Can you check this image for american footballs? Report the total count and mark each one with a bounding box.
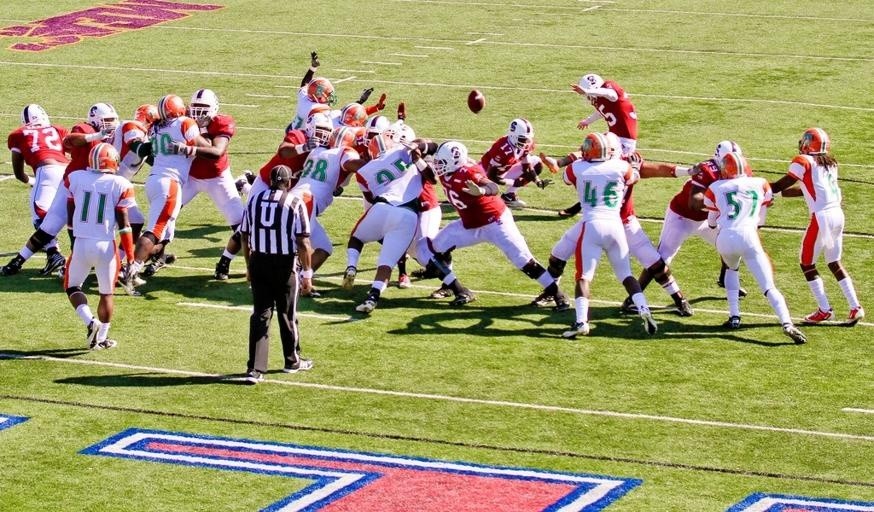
[469,90,484,115]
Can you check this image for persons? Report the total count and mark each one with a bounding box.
[1,52,749,338]
[704,152,808,345]
[769,127,864,328]
[63,142,135,350]
[240,164,313,384]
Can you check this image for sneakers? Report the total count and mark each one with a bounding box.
[559,210,572,216]
[501,192,526,208]
[234,171,254,193]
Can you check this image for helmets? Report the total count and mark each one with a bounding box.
[579,74,605,91]
[87,104,117,134]
[21,104,50,128]
[509,119,532,151]
[581,132,621,162]
[712,140,754,177]
[133,89,219,132]
[88,142,119,173]
[799,128,829,155]
[307,77,469,172]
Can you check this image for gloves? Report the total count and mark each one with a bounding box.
[463,179,487,198]
[168,139,197,157]
[310,51,319,71]
[333,187,344,197]
[536,179,554,188]
[687,162,709,178]
[98,128,115,140]
[628,152,641,169]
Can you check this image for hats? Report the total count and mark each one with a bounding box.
[270,165,297,181]
